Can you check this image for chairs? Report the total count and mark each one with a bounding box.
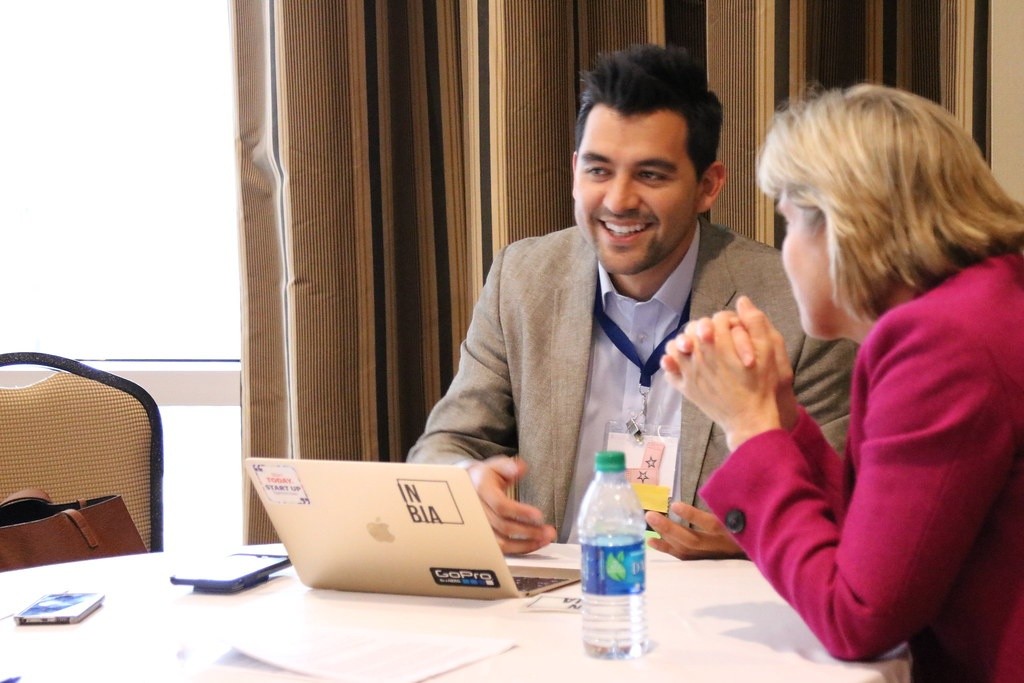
[0,351,165,551]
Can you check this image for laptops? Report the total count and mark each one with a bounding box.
[245,456,582,600]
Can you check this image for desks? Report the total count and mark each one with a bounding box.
[0,543,914,683]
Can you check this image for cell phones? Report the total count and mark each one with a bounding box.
[14,591,107,626]
[171,553,292,594]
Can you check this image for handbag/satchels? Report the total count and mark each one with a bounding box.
[0,489,148,573]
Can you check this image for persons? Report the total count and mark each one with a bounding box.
[406,43,858,562]
[660,85,1023,683]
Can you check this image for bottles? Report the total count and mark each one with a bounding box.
[576,450,651,661]
